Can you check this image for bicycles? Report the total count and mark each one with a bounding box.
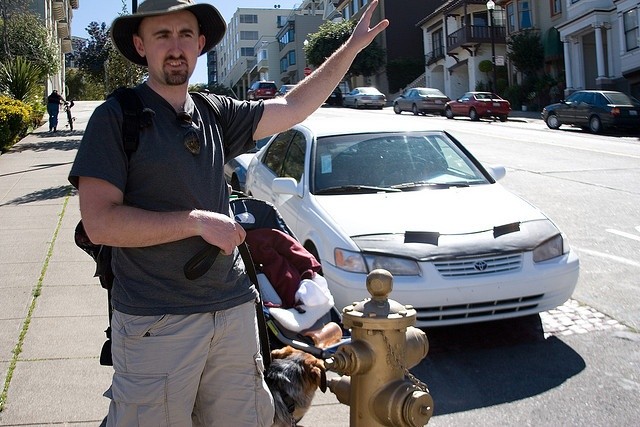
[59,101,76,131]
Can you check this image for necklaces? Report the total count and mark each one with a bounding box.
[178,110,201,154]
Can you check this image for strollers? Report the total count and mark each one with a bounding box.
[224,190,350,376]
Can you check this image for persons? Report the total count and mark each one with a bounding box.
[47,88,66,132]
[68,1,389,427]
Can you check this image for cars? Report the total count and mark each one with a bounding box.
[324,268,434,426]
[322,86,343,107]
[247,81,277,101]
[444,92,511,122]
[275,85,297,100]
[393,88,450,116]
[541,91,640,134]
[246,119,579,336]
[342,87,386,110]
[222,130,279,198]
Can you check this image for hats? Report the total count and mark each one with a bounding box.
[109,0,227,66]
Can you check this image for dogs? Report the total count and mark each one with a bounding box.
[100,336,327,427]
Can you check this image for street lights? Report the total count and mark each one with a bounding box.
[486,0,499,95]
